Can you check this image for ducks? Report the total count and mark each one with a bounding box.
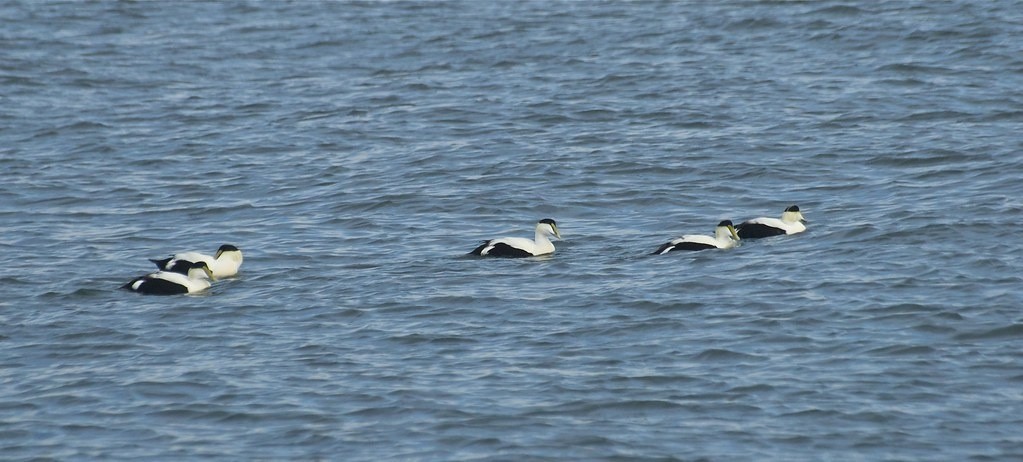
[649,205,807,256]
[469,218,561,257]
[115,244,243,292]
